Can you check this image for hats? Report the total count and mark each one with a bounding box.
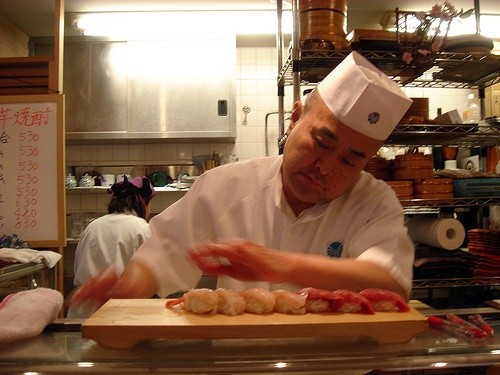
[317,51,414,141]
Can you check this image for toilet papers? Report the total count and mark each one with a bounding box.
[409,217,466,250]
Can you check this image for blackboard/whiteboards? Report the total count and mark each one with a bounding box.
[0,93,68,248]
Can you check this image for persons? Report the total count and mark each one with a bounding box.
[66,176,156,318]
[69,52,416,317]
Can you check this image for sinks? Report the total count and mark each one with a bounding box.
[167,181,193,189]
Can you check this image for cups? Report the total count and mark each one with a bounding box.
[99,174,115,186]
[70,212,97,239]
[151,170,167,187]
[117,174,130,184]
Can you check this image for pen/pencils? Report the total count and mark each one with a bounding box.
[428,314,495,340]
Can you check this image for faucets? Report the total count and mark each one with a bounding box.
[179,172,190,177]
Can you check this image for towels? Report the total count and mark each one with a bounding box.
[1,245,62,270]
[0,286,66,341]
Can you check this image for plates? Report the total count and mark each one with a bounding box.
[457,229,500,280]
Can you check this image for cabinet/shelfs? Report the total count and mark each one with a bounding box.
[29,32,237,142]
[276,0,500,289]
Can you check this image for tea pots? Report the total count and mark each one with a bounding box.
[79,173,97,187]
[65,173,77,188]
